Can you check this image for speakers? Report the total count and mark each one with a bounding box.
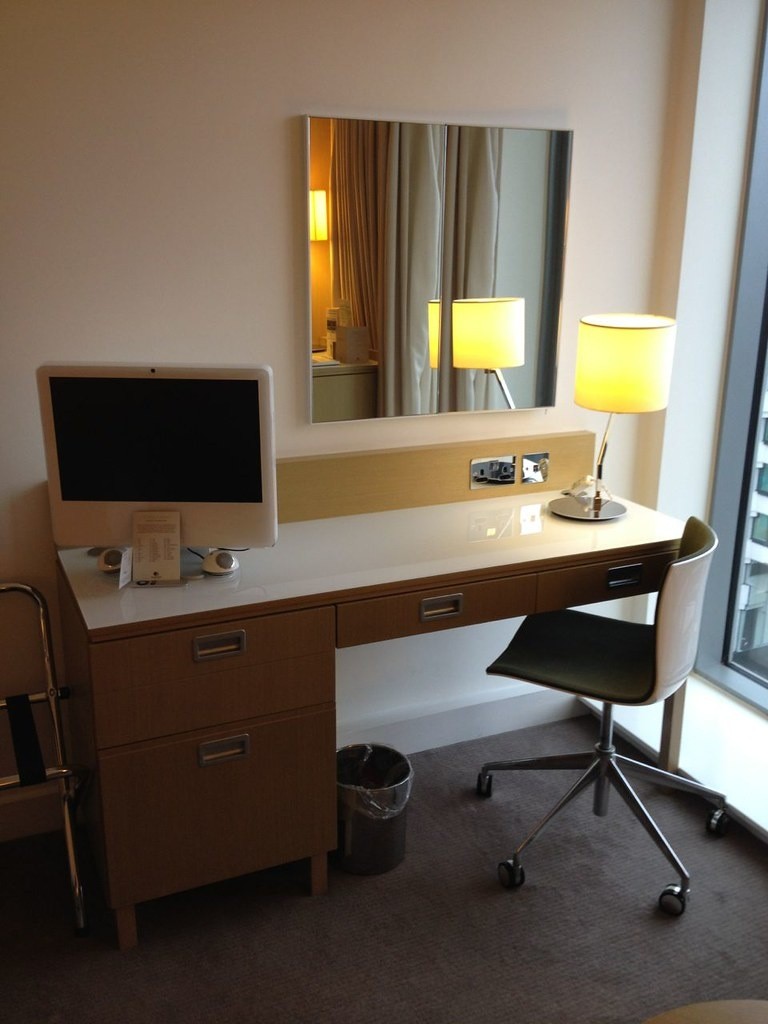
[200,549,239,576]
[98,548,124,574]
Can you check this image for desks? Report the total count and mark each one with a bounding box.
[49,538,697,954]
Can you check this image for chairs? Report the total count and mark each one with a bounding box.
[474,516,730,917]
[0,582,83,938]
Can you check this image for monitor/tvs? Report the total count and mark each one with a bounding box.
[36,363,279,580]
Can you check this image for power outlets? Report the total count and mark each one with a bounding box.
[469,451,548,491]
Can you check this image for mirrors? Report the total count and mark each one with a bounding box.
[303,117,572,428]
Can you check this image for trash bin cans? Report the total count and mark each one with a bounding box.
[335,743,415,876]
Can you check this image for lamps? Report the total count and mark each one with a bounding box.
[550,312,679,522]
[453,299,534,412]
